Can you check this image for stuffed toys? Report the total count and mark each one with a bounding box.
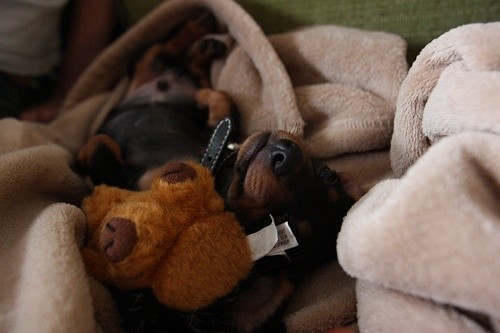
[82,161,253,311]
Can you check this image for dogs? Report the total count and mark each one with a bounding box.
[73,9,360,286]
